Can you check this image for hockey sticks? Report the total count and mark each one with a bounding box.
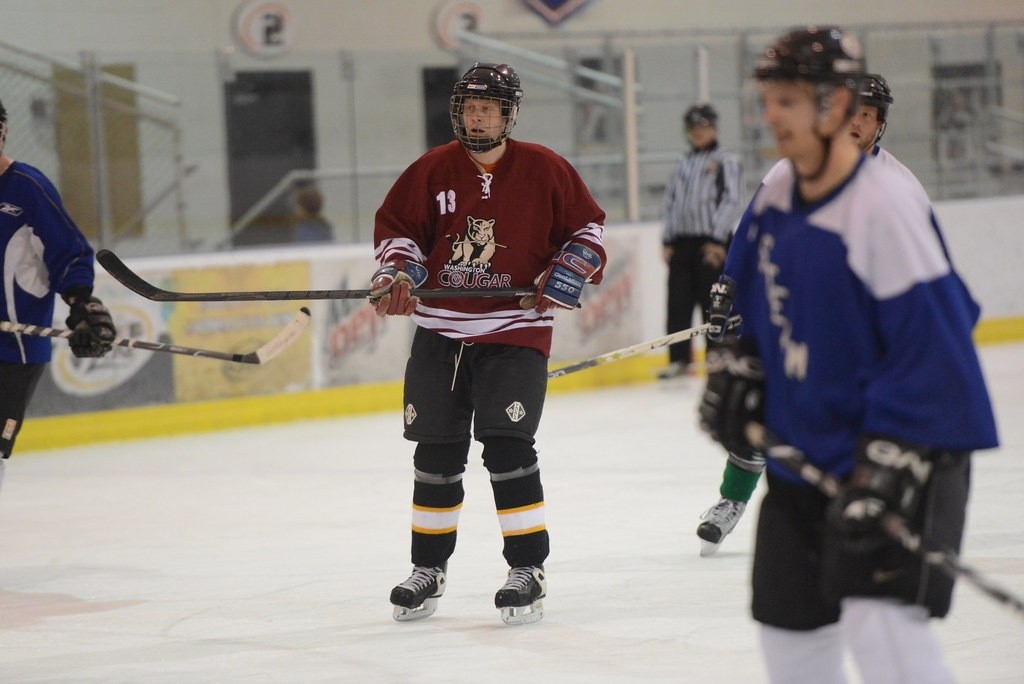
[0,308,311,366]
[548,314,736,380]
[740,412,1024,623]
[95,247,578,307]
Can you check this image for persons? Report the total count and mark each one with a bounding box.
[293,188,332,243]
[701,25,1001,684]
[698,74,981,553]
[0,100,117,485]
[370,62,608,624]
[659,103,743,379]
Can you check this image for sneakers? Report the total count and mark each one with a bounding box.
[390,562,447,621]
[697,498,746,555]
[495,566,547,626]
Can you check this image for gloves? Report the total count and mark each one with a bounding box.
[65,297,116,359]
[531,242,601,314]
[368,259,427,317]
[662,246,672,263]
[696,357,764,444]
[840,438,931,543]
[704,243,726,266]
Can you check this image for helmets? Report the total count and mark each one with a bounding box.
[0,102,8,151]
[858,73,894,106]
[747,24,867,86]
[449,63,524,152]
[684,103,718,126]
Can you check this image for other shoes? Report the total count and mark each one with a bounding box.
[659,362,686,378]
